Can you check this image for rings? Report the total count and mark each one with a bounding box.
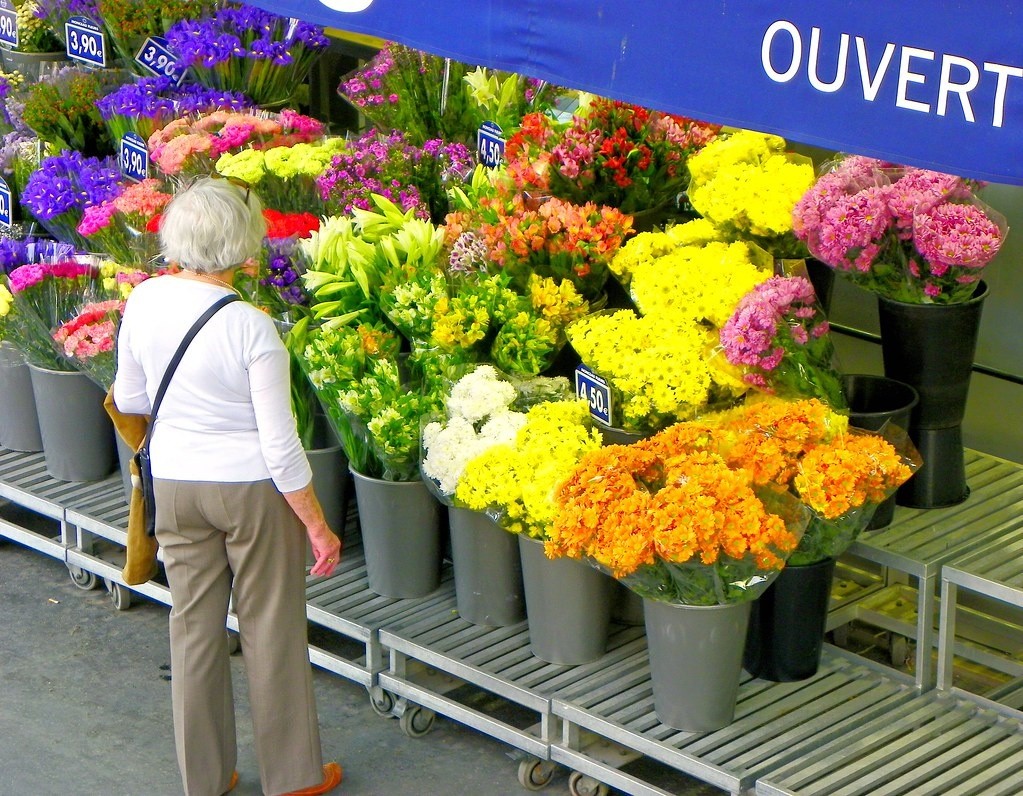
[325,559,334,563]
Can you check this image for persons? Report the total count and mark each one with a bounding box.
[114,175,341,796]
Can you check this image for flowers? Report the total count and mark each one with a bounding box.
[686,129,814,259]
[682,393,924,568]
[791,151,1008,305]
[454,396,606,541]
[717,275,849,412]
[563,307,712,431]
[419,366,528,503]
[542,421,814,607]
[0,0,719,479]
[607,217,773,403]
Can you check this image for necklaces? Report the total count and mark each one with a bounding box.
[183,267,245,301]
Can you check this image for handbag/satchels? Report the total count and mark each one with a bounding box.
[133,446,155,535]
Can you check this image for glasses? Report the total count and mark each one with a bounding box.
[187,172,251,208]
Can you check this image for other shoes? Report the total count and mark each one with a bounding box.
[225,769,238,792]
[278,762,342,796]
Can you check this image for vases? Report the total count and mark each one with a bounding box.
[112,424,138,506]
[642,597,753,733]
[75,58,128,79]
[0,341,44,452]
[893,425,971,510]
[347,459,445,598]
[447,507,527,627]
[777,256,836,320]
[518,533,615,665]
[1,47,73,79]
[610,580,648,626]
[739,556,836,684]
[837,374,919,532]
[128,66,145,82]
[84,250,117,261]
[876,280,992,430]
[25,360,115,481]
[18,232,57,246]
[260,92,299,113]
[302,413,348,565]
[271,318,322,336]
